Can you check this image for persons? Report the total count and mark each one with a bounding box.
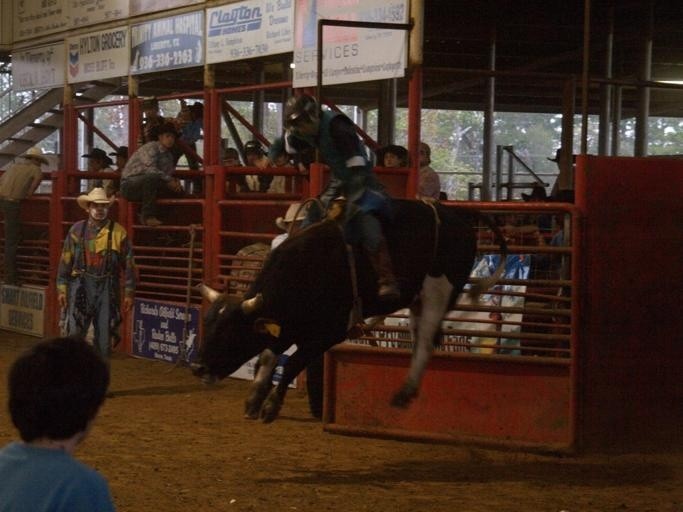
[376,143,408,169]
[0,332,116,511]
[280,92,404,303]
[546,148,577,197]
[56,187,139,398]
[464,185,576,304]
[0,147,50,285]
[419,142,442,200]
[119,121,185,227]
[80,95,310,197]
[269,201,309,252]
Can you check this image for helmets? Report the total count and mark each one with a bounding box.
[282,93,317,126]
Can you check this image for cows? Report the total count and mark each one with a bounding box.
[188,193,508,425]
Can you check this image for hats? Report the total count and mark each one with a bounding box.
[77,187,116,209]
[156,122,179,138]
[109,146,129,158]
[81,148,113,165]
[374,145,409,163]
[20,148,49,166]
